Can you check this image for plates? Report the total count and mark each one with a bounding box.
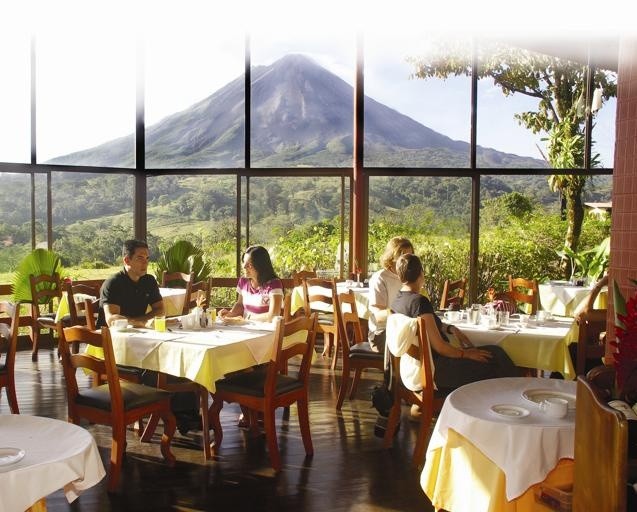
[489,403,531,418]
[0,446,25,468]
[521,387,577,410]
[548,280,570,285]
[129,331,188,342]
[249,322,275,332]
[110,325,134,331]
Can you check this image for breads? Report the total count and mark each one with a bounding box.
[166,319,178,323]
[224,316,243,324]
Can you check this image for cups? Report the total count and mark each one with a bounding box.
[539,396,569,419]
[111,319,127,329]
[570,274,588,285]
[443,304,554,330]
[179,306,217,328]
[154,315,165,331]
[345,279,353,287]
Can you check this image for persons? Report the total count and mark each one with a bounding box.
[390,253,520,388]
[83,239,204,436]
[216,245,285,427]
[366,235,415,353]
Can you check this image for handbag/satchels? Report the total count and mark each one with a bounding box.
[374,415,401,437]
[481,300,515,316]
[370,385,401,417]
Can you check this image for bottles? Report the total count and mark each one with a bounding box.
[363,279,370,288]
[63,276,71,291]
